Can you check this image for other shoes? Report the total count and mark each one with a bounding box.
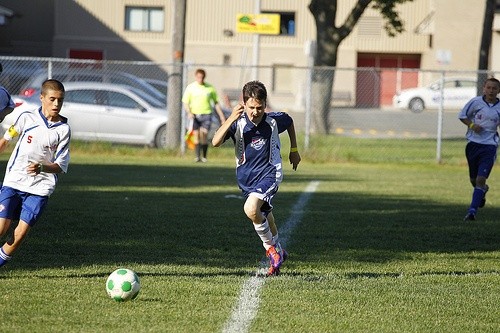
[194,158,207,163]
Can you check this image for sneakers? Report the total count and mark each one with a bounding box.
[464,211,476,221]
[479,184,489,208]
[266,242,289,277]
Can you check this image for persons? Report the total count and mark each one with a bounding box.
[0,79,71,269]
[211,80,302,277]
[0,62,15,123]
[458,78,500,220]
[181,68,226,163]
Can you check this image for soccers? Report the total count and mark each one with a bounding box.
[106,268,141,302]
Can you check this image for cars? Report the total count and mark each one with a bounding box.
[11,80,220,150]
[393,75,478,113]
[19,67,168,104]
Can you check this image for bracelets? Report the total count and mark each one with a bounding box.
[39,163,44,172]
[290,147,298,152]
[469,123,475,129]
